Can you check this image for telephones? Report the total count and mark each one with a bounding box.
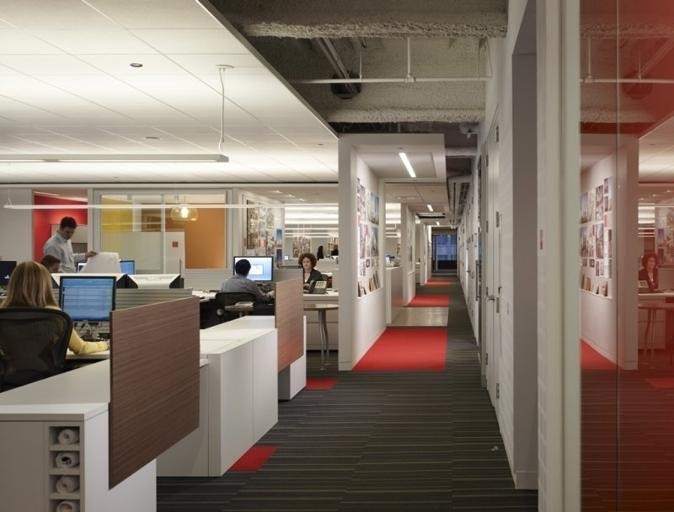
[308,280,327,294]
[638,279,652,294]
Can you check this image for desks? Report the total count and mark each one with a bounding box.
[633,301,674,375]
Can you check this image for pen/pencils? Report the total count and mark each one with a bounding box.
[74,321,85,334]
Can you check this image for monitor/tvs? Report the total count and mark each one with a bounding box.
[78,263,86,272]
[60,275,117,323]
[233,256,274,282]
[0,261,17,279]
[119,260,135,275]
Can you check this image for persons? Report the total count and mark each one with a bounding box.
[316,245,324,261]
[39,254,61,273]
[295,252,323,292]
[330,245,338,256]
[215,258,275,303]
[657,248,670,261]
[0,259,111,356]
[639,253,661,292]
[42,216,97,273]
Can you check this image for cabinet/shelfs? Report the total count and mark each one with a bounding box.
[638,290,673,354]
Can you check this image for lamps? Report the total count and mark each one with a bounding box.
[0,62,238,168]
[170,192,199,224]
